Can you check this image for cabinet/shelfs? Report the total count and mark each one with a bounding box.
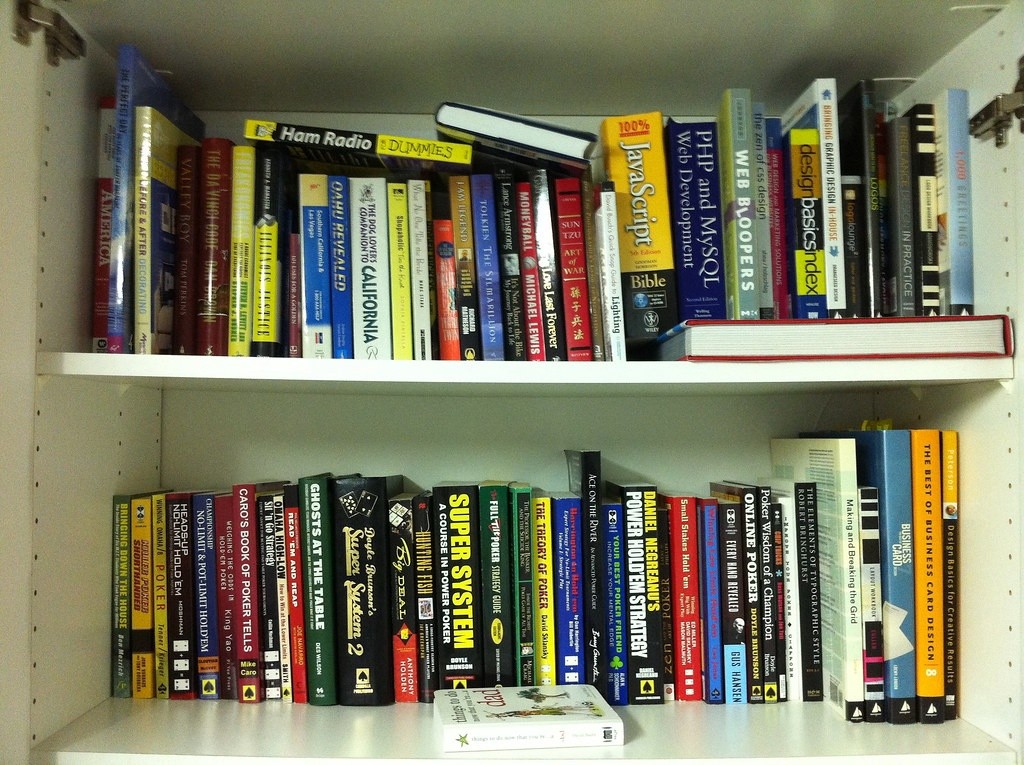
[0,0,1024,765]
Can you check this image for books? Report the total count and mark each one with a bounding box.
[91,44,1013,753]
